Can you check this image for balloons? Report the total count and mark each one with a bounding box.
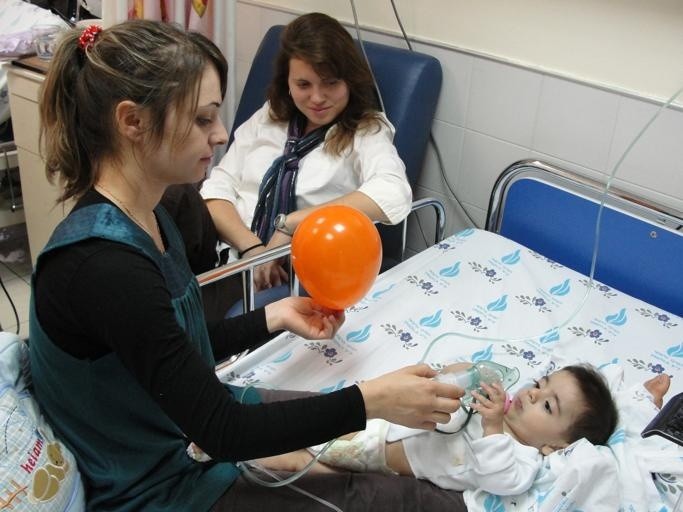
[292,206,383,314]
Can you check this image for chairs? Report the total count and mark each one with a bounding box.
[223,21,444,321]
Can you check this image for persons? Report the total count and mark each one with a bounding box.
[236,364,618,496]
[27,21,670,512]
[162,12,413,327]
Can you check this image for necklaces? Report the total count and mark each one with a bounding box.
[93,179,168,254]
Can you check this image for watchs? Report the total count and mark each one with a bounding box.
[273,212,294,237]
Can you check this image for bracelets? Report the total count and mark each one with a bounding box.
[237,242,265,257]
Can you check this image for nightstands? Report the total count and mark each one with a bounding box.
[10,67,84,272]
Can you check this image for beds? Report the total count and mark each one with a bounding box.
[195,160,683,512]
